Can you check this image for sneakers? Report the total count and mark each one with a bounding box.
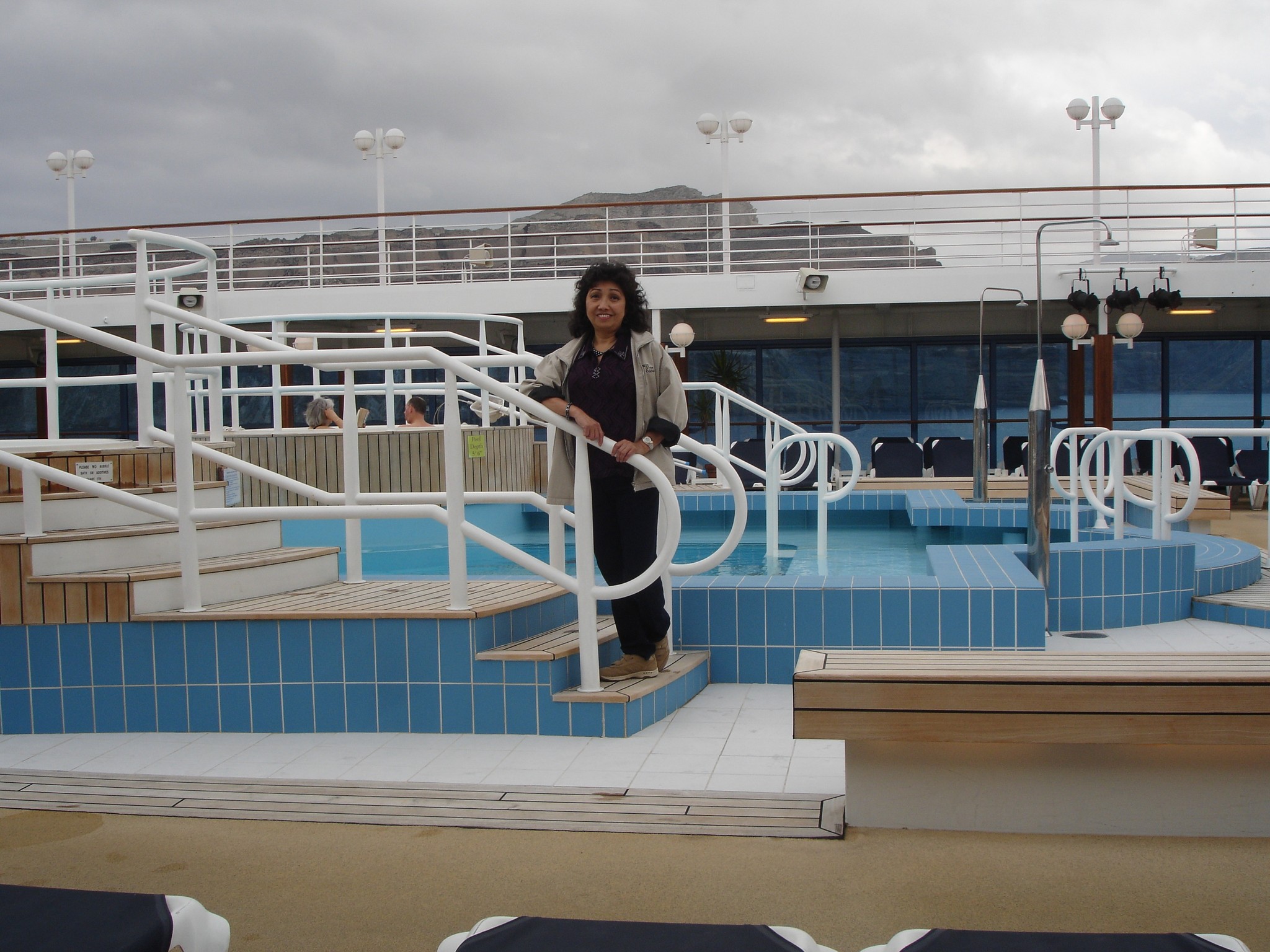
[599,654,659,681]
[654,633,670,672]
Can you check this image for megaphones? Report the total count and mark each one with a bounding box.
[469,244,493,267]
[177,287,204,311]
[796,268,829,294]
[1193,225,1218,250]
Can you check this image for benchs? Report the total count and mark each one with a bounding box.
[795,647,1270,839]
[1123,477,1231,531]
[839,478,1118,497]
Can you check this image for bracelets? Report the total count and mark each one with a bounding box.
[565,403,572,421]
[335,418,341,424]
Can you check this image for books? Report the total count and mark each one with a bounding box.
[356,407,369,428]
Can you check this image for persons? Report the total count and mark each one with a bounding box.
[306,395,436,430]
[518,259,688,680]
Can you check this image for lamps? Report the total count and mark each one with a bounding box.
[1112,313,1144,349]
[1104,267,1141,315]
[1147,266,1182,310]
[1067,268,1101,312]
[367,320,417,332]
[292,338,311,366]
[1181,225,1217,254]
[246,342,271,367]
[1060,314,1093,350]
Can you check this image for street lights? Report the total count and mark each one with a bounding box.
[351,127,406,285]
[696,111,752,274]
[1064,94,1125,265]
[45,147,95,297]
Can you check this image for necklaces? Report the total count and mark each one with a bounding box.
[593,340,617,356]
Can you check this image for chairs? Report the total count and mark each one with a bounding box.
[1004,435,1270,515]
[871,436,976,479]
[673,452,696,485]
[0,889,1252,952]
[730,439,835,492]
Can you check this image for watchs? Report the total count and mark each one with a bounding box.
[640,435,654,451]
